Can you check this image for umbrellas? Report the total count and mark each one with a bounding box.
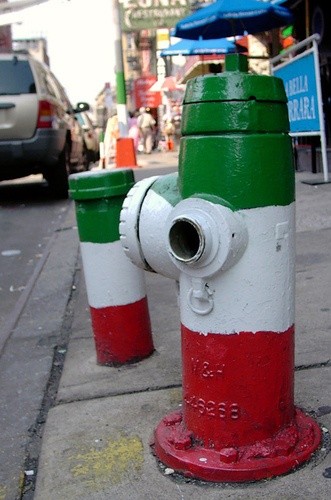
[161,35,249,77]
[170,1,295,54]
[150,77,191,92]
[176,54,227,85]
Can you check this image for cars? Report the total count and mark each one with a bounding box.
[77,111,100,162]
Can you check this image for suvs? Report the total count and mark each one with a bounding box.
[0,52,91,196]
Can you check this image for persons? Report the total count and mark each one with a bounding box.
[104,112,120,168]
[129,111,143,154]
[137,107,158,155]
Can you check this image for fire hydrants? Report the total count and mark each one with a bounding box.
[118,53,322,481]
